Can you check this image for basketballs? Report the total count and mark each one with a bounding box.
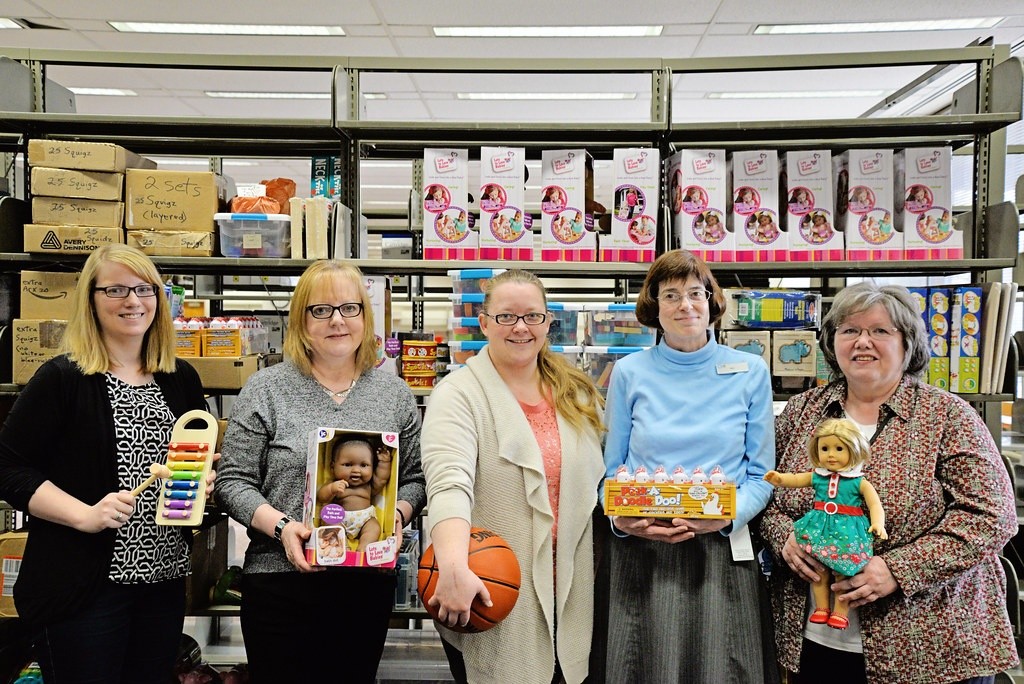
[418,526,520,634]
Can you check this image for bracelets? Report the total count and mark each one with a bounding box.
[395,507,406,528]
[274,516,297,542]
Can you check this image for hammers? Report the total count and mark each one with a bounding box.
[129,463,172,495]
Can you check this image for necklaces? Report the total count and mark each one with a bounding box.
[310,370,355,398]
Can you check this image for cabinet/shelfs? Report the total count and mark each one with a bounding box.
[0,42,1024,684]
[952,56,1024,659]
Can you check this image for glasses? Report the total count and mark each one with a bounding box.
[834,322,903,340]
[305,300,364,319]
[656,289,712,306]
[94,284,160,298]
[479,311,548,325]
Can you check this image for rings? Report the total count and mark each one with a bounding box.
[117,512,123,519]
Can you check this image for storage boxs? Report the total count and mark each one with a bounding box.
[25,139,292,258]
[362,274,400,378]
[447,270,657,390]
[0,530,28,618]
[719,288,983,393]
[302,427,399,569]
[605,475,737,519]
[392,530,419,611]
[218,416,228,454]
[423,147,964,261]
[14,269,279,389]
[186,516,229,609]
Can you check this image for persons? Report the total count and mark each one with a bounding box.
[480,183,523,239]
[904,184,950,237]
[424,185,466,239]
[215,261,428,684]
[419,271,610,684]
[0,241,223,684]
[598,246,776,684]
[316,437,393,553]
[680,188,725,243]
[762,419,888,630]
[757,283,1021,684]
[622,187,655,242]
[735,187,778,244]
[849,188,892,240]
[788,187,833,242]
[321,529,342,558]
[542,187,585,241]
